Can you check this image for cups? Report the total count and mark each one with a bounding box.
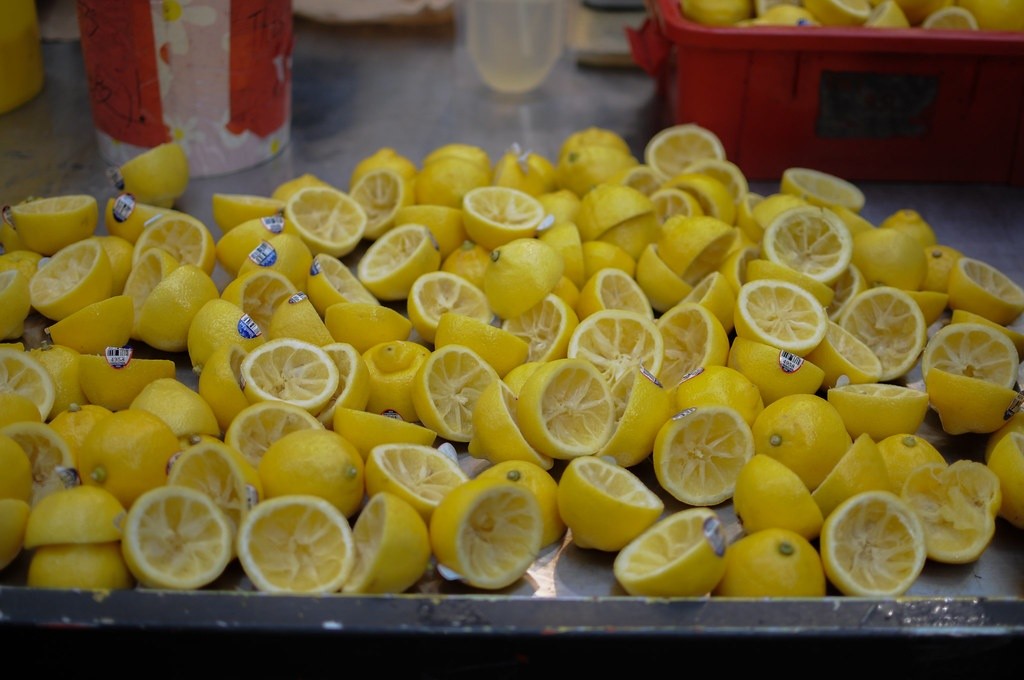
[79,0,292,178]
[467,0,561,92]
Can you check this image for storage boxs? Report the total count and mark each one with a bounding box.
[639,0,1024,184]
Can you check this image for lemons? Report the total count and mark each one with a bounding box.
[679,0,1024,30]
[0,125,1024,597]
